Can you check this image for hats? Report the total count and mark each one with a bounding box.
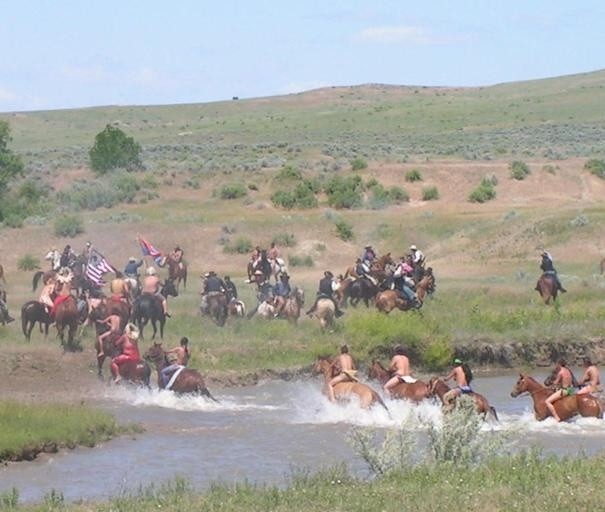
[254,270,264,275]
[409,245,417,250]
[145,266,156,275]
[364,244,371,248]
[280,272,289,277]
[324,271,333,277]
[207,271,216,275]
[541,253,548,256]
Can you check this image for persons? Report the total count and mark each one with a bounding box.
[534,253,568,293]
[96,307,120,358]
[544,360,573,422]
[328,346,356,401]
[443,359,471,404]
[577,357,600,394]
[161,338,191,387]
[111,323,140,383]
[382,346,409,392]
[2,245,427,323]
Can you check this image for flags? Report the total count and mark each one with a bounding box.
[140,239,161,256]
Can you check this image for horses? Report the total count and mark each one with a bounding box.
[142,341,218,403]
[544,363,605,411]
[199,252,436,334]
[310,351,392,420]
[536,272,558,305]
[367,358,432,406]
[511,372,604,424]
[426,375,500,423]
[20,247,187,388]
